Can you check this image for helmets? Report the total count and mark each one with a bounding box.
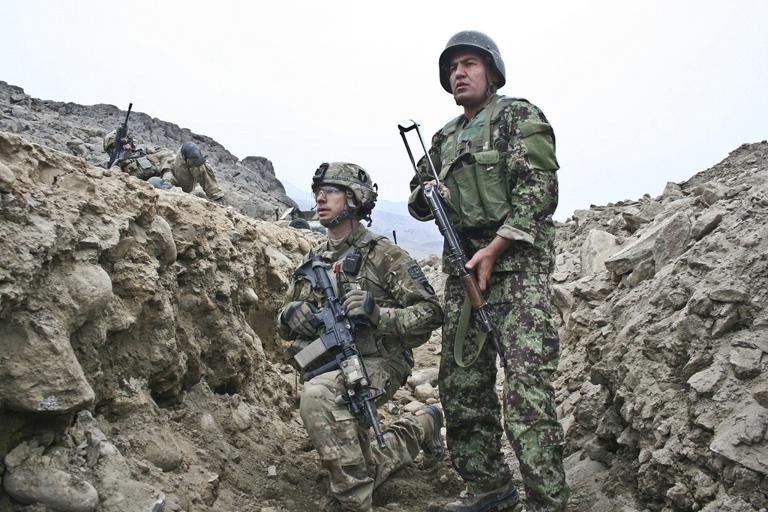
[312,161,378,209]
[104,130,129,149]
[438,31,505,93]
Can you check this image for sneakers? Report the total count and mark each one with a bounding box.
[446,480,519,512]
[408,406,444,468]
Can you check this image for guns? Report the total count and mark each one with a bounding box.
[398,122,506,368]
[292,250,386,451]
[107,104,132,168]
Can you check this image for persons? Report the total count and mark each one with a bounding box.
[407,30,570,512]
[274,161,445,512]
[101,129,230,207]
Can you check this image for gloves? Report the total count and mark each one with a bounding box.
[340,290,379,328]
[284,301,320,339]
